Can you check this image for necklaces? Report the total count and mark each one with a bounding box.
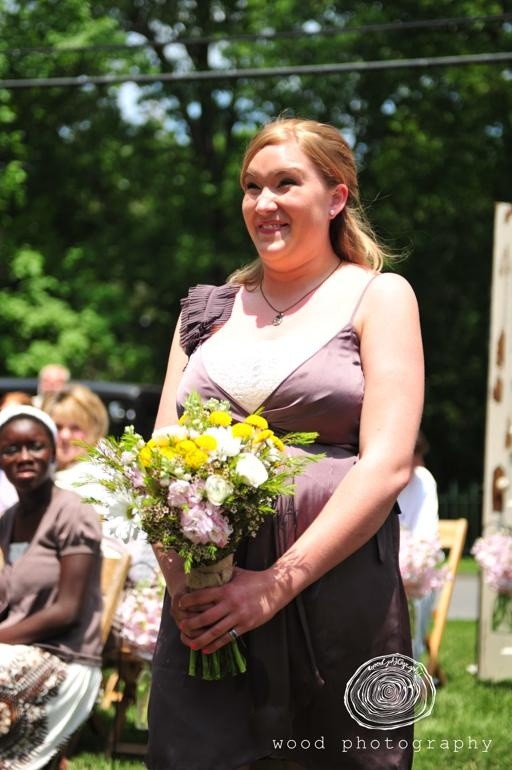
[260,259,342,326]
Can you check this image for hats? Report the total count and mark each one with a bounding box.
[0,404,57,438]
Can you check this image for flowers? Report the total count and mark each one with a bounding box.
[470,529,512,633]
[71,391,326,686]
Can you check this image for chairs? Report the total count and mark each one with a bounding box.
[55,535,147,769]
[420,518,470,689]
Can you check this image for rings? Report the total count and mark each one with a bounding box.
[228,628,238,640]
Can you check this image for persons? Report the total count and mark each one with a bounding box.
[397,432,445,690]
[144,109,425,770]
